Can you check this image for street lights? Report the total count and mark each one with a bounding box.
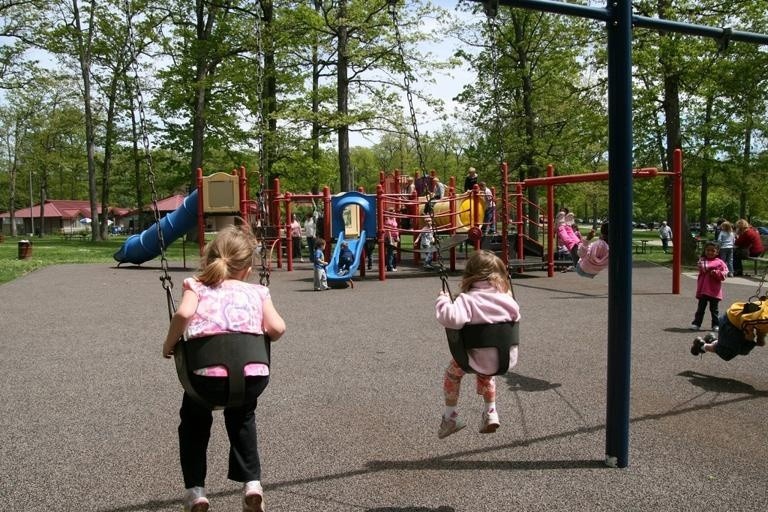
[29,168,38,235]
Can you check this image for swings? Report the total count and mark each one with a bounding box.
[388,1,520,375]
[568,27,761,277]
[742,269,768,343]
[122,1,270,409]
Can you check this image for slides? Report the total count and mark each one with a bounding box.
[326,231,366,282]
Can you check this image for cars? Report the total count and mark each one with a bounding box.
[540,215,608,226]
[757,227,768,235]
[632,220,718,233]
[61,220,92,235]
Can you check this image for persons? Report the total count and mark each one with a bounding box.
[384,207,400,271]
[414,218,434,270]
[386,168,445,228]
[479,182,496,236]
[659,221,672,253]
[464,168,478,192]
[293,212,323,262]
[689,294,768,363]
[715,219,766,276]
[687,239,729,333]
[314,239,354,291]
[434,249,521,438]
[163,223,285,511]
[357,186,373,269]
[556,209,609,278]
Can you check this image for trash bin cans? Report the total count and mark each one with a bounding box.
[18,240,32,260]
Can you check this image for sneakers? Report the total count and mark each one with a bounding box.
[688,324,700,331]
[184,486,210,512]
[712,326,719,332]
[437,411,467,439]
[556,212,565,224]
[242,480,266,512]
[478,408,501,434]
[691,335,706,356]
[566,212,574,224]
[703,332,718,344]
